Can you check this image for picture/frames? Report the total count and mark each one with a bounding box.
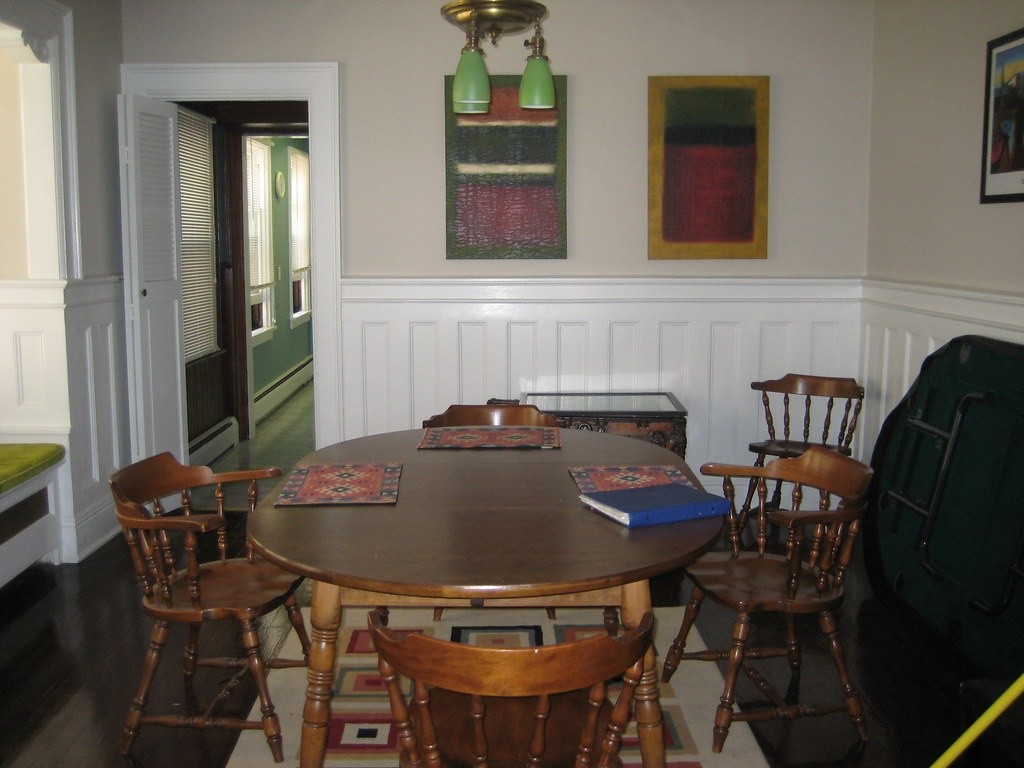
[444,74,567,261]
[647,75,769,261]
[979,26,1024,204]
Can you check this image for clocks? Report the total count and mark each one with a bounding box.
[276,171,286,198]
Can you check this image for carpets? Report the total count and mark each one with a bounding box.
[222,608,773,768]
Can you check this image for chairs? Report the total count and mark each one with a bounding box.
[423,404,557,622]
[662,446,877,754]
[365,608,657,768]
[107,452,309,765]
[730,372,865,557]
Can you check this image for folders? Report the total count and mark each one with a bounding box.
[578,483,731,529]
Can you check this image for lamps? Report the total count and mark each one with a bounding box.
[440,0,556,113]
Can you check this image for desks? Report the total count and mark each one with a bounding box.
[518,391,687,462]
[245,425,724,768]
